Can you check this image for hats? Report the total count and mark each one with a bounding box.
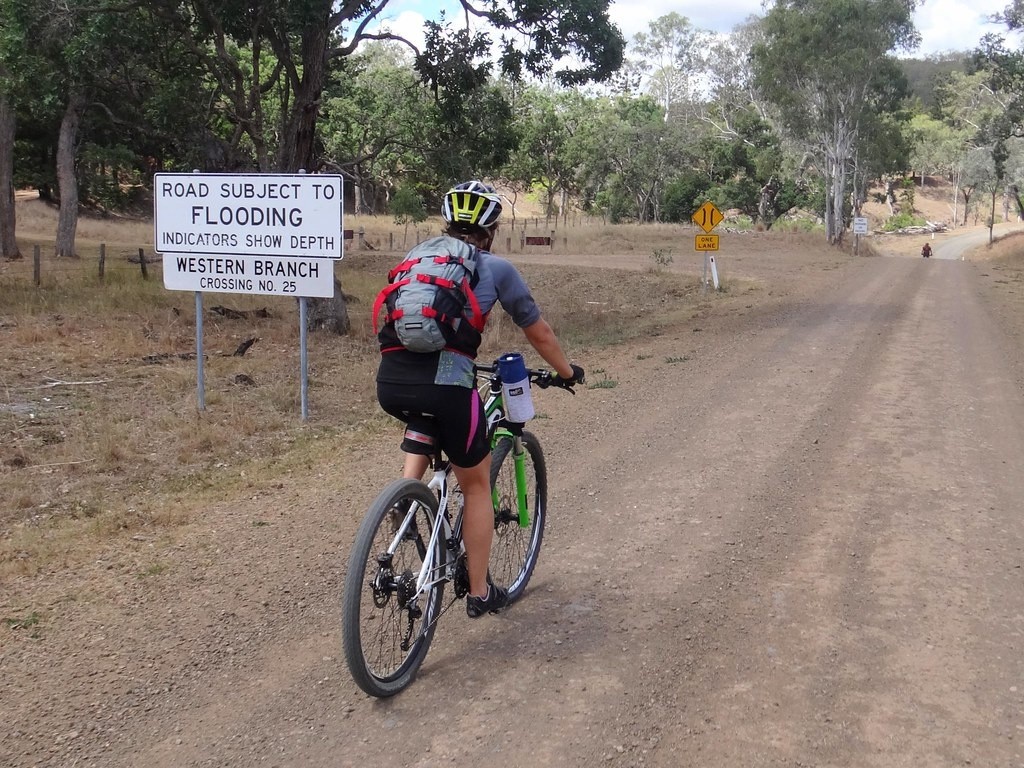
[441,182,503,227]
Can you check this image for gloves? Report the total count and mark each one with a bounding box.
[569,364,585,385]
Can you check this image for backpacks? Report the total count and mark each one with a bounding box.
[373,235,482,355]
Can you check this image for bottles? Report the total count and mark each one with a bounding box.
[499,352,534,423]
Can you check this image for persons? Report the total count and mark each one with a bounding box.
[922,243,932,258]
[376,181,584,619]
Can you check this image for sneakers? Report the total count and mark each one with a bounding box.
[391,496,419,537]
[467,584,508,617]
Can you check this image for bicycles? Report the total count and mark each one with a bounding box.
[336,363,585,696]
[921,253,932,258]
